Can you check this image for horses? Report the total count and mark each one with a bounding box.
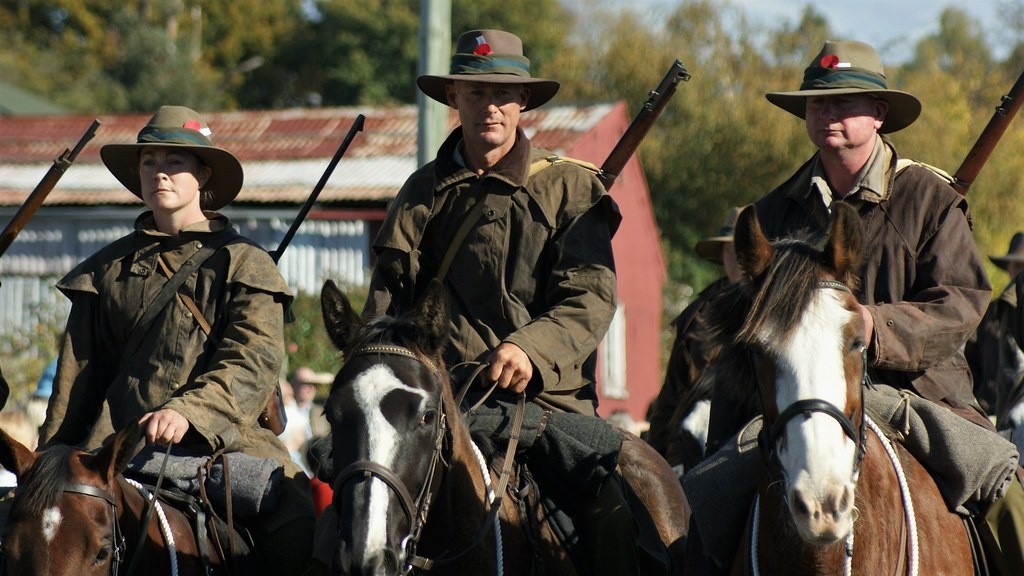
[0,200,995,576]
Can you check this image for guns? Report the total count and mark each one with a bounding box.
[949,73,1024,195]
[594,56,694,193]
[269,111,369,263]
[1,117,101,253]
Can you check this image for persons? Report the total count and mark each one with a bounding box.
[34,105,331,567]
[730,41,1023,572]
[360,28,624,420]
[281,365,335,468]
[674,219,743,328]
[968,229,1023,416]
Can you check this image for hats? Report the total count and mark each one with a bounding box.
[765,40,921,134]
[417,29,560,113]
[988,232,1024,269]
[100,106,244,211]
